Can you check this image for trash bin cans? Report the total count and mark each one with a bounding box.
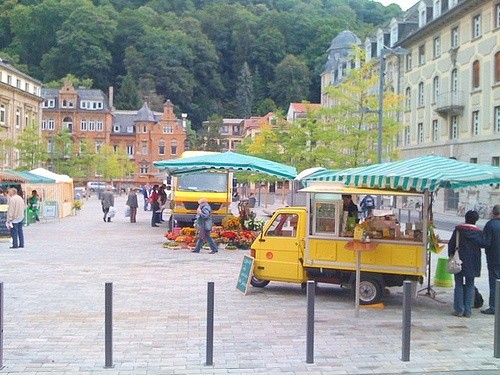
[249,198,255,208]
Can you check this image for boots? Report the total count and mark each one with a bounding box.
[108,217,110,222]
[103,215,106,222]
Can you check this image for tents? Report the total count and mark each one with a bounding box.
[154,149,297,252]
[0,167,74,226]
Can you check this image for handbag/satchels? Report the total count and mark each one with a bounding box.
[454,284,483,308]
[111,207,116,218]
[446,250,462,273]
[125,208,130,217]
[147,191,156,204]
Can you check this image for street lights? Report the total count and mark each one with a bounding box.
[46,131,63,172]
[357,46,411,211]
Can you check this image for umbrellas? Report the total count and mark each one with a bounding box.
[303,152,500,299]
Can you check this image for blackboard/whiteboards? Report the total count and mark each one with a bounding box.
[236,254,254,295]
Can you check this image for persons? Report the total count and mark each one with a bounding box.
[482,204,500,314]
[191,197,218,254]
[448,210,482,319]
[101,185,115,223]
[4,188,9,204]
[6,188,25,249]
[0,187,7,205]
[342,194,358,224]
[124,182,167,228]
[29,190,40,223]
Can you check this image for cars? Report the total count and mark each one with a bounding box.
[151,185,172,201]
[74,187,91,198]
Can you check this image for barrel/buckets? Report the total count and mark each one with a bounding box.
[434,258,454,288]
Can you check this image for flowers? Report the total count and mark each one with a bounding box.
[164,216,265,251]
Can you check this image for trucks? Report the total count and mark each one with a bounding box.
[250,183,430,305]
[167,150,238,231]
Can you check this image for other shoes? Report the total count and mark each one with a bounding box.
[209,249,218,254]
[152,225,159,227]
[159,220,164,222]
[10,246,24,248]
[192,251,199,253]
[487,309,496,315]
[155,222,162,224]
[451,312,470,317]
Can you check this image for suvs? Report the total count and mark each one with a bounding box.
[87,181,115,192]
